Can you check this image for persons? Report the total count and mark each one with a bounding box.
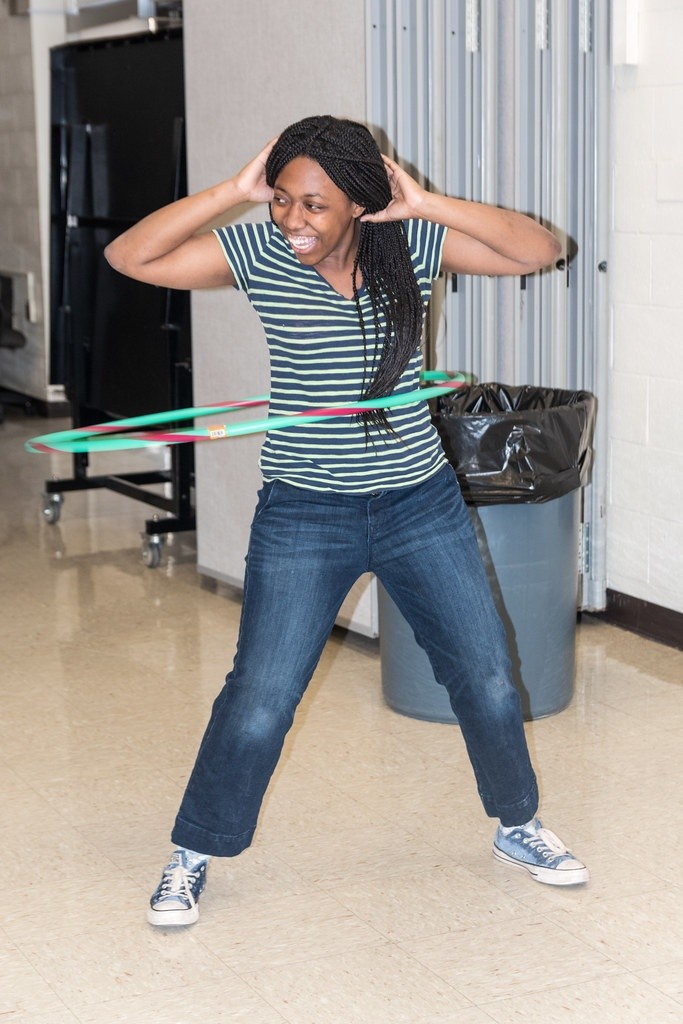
[101,114,590,929]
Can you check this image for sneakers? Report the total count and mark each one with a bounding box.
[146,850,208,926]
[492,817,590,885]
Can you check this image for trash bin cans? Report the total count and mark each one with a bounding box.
[377,380,598,725]
